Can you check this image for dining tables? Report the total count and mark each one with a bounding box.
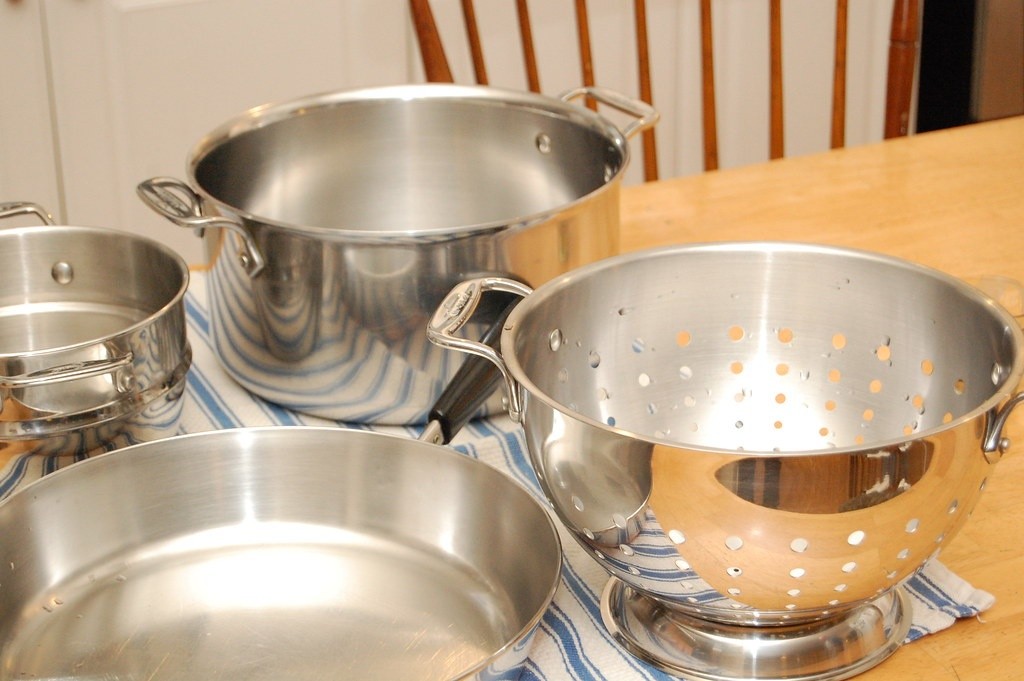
[0,116,1024,681]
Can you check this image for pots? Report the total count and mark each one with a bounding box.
[0,202,192,456]
[135,82,660,425]
[0,295,562,681]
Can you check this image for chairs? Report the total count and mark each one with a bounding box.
[411,0,922,182]
